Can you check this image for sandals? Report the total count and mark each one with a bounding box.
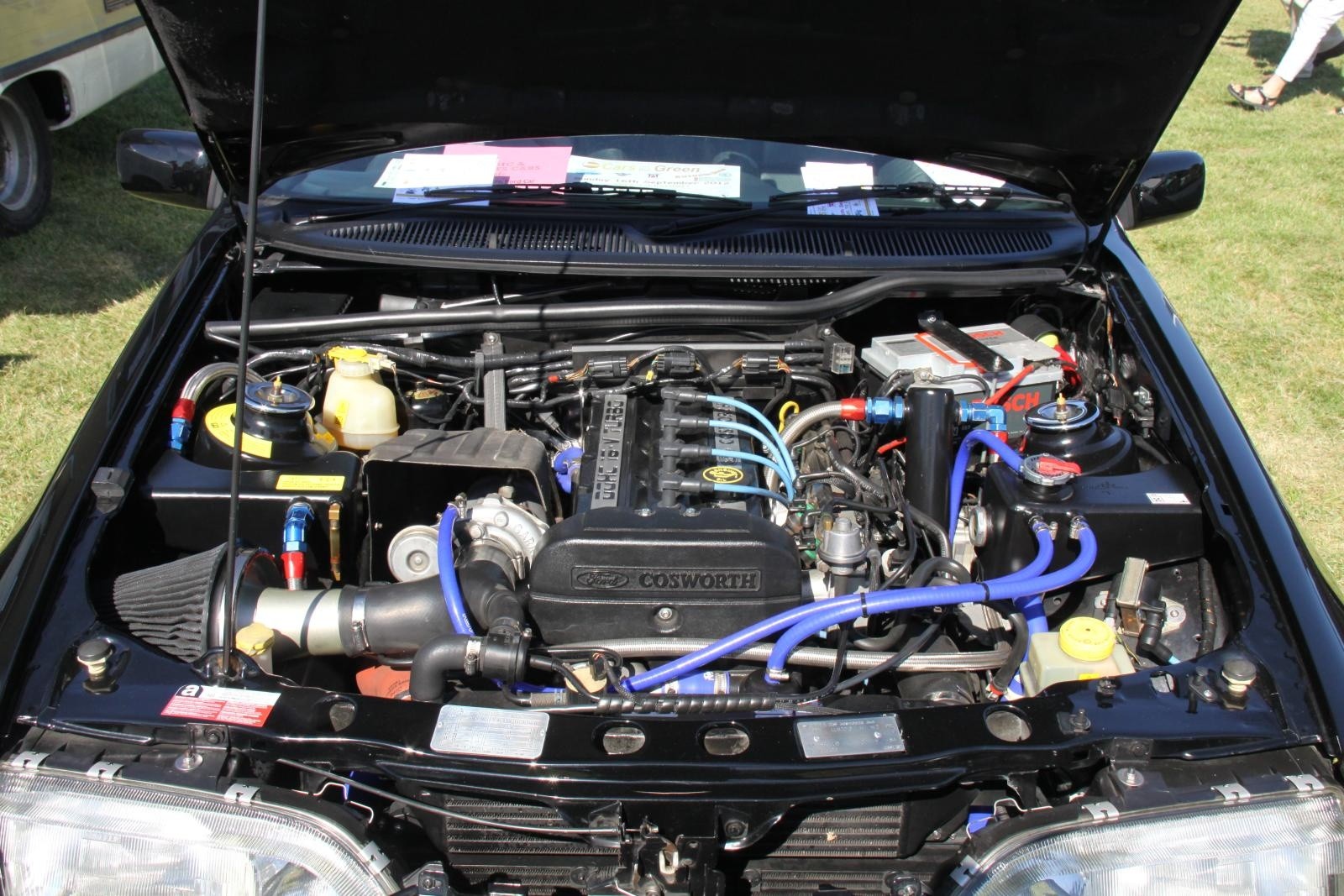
[1227,83,1279,111]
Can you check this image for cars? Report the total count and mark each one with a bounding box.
[2,0,1343,896]
[0,0,168,236]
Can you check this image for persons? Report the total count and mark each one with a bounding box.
[1227,0,1344,110]
